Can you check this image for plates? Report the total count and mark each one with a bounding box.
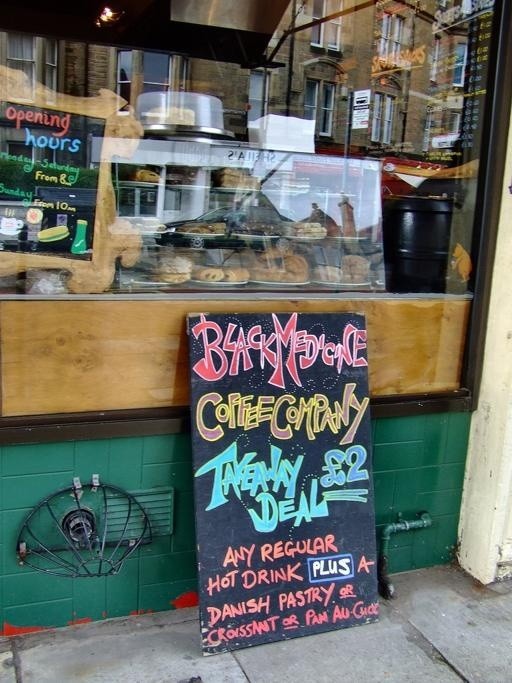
[259,187,309,197]
[164,184,212,192]
[231,232,281,241]
[110,180,162,190]
[250,278,311,288]
[281,236,325,241]
[214,185,256,195]
[137,232,165,239]
[190,280,249,287]
[316,192,357,199]
[312,280,373,287]
[327,236,369,241]
[132,280,174,288]
[167,230,227,241]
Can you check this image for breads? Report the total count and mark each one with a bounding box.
[147,247,371,285]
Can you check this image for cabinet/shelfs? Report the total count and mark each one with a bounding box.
[86,135,383,295]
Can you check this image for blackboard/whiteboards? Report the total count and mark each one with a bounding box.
[186,311,380,657]
[367,0,493,186]
[0,101,106,261]
